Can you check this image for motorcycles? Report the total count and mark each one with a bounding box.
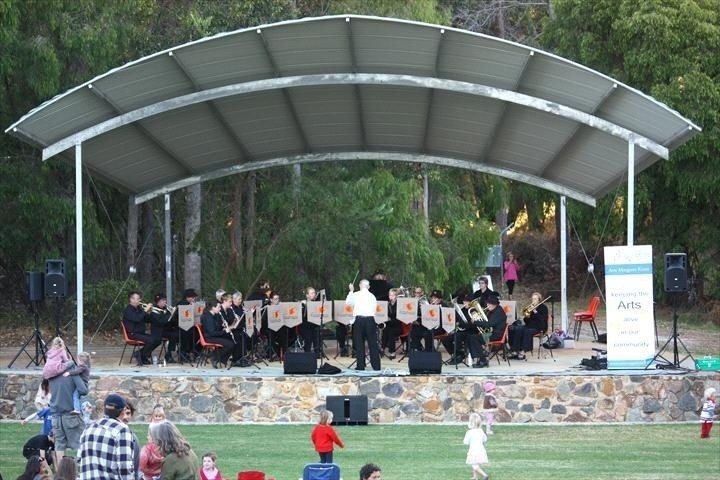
[8,300,48,368]
[26,298,77,368]
[644,292,695,370]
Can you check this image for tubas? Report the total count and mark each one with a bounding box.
[468,297,494,334]
[448,294,468,331]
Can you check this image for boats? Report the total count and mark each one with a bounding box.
[326,396,368,424]
[44,258,68,297]
[663,253,688,291]
[26,271,45,300]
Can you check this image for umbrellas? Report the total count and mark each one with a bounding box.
[472,360,489,369]
[355,366,365,370]
[210,357,218,369]
[142,357,151,365]
[164,353,176,363]
[133,350,143,366]
[507,353,525,360]
[390,352,396,359]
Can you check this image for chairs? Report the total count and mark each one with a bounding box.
[69,410,81,415]
[469,474,489,480]
[485,430,494,436]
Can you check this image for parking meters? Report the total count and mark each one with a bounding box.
[517,295,550,321]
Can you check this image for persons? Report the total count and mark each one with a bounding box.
[483,382,499,434]
[464,413,489,477]
[16,337,223,478]
[123,280,322,369]
[336,273,508,371]
[699,387,717,439]
[360,463,381,478]
[504,251,520,300]
[507,292,547,360]
[311,410,344,463]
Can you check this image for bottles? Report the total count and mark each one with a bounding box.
[485,381,497,393]
[703,387,716,398]
[103,394,127,410]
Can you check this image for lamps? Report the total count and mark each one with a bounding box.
[571,293,605,344]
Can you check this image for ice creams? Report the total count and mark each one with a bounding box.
[138,302,167,315]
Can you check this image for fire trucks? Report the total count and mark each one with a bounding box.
[304,291,320,307]
